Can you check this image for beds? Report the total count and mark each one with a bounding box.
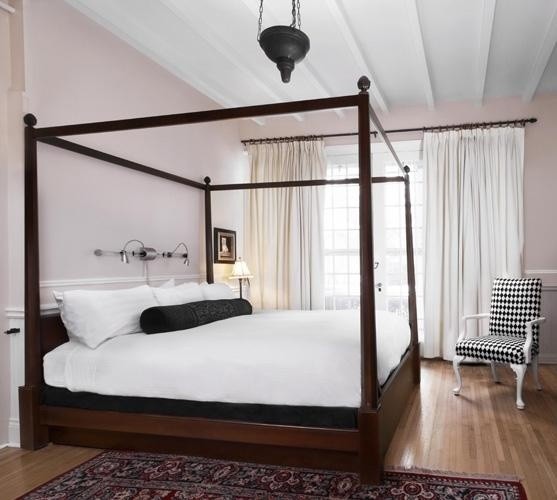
[20,76,424,472]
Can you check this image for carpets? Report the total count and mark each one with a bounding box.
[18,449,526,499]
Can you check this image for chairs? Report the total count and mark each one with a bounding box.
[452,277,546,409]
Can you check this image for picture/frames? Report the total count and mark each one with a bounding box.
[212,228,236,263]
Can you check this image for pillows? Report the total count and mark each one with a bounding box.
[52,277,254,349]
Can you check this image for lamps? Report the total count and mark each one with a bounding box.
[87,239,189,283]
[228,254,252,302]
[254,1,310,84]
